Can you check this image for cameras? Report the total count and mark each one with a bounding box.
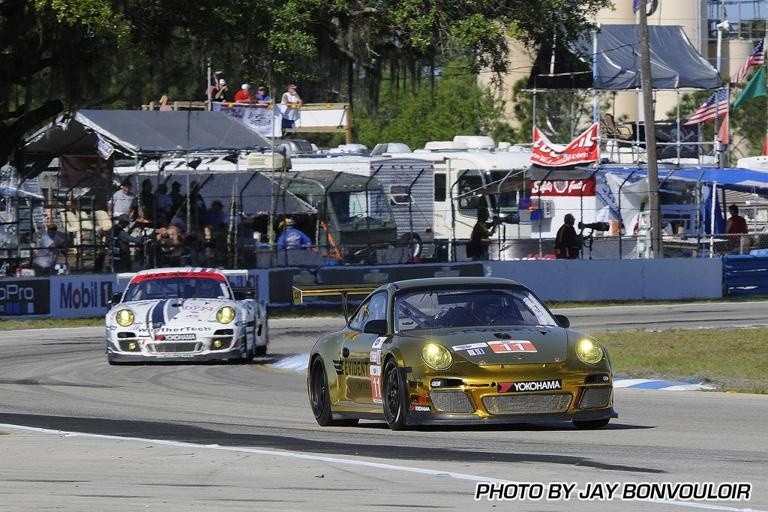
[161,233,170,239]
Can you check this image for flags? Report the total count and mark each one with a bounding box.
[684,84,728,125]
[731,35,767,83]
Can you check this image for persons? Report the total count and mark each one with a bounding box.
[411,232,423,258]
[471,208,497,260]
[281,84,304,128]
[254,87,272,101]
[31,224,73,277]
[725,204,748,244]
[276,217,312,250]
[214,79,229,102]
[224,85,233,102]
[553,214,583,259]
[107,179,255,268]
[232,84,256,103]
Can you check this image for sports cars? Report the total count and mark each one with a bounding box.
[306,276,618,430]
[105,266,268,364]
